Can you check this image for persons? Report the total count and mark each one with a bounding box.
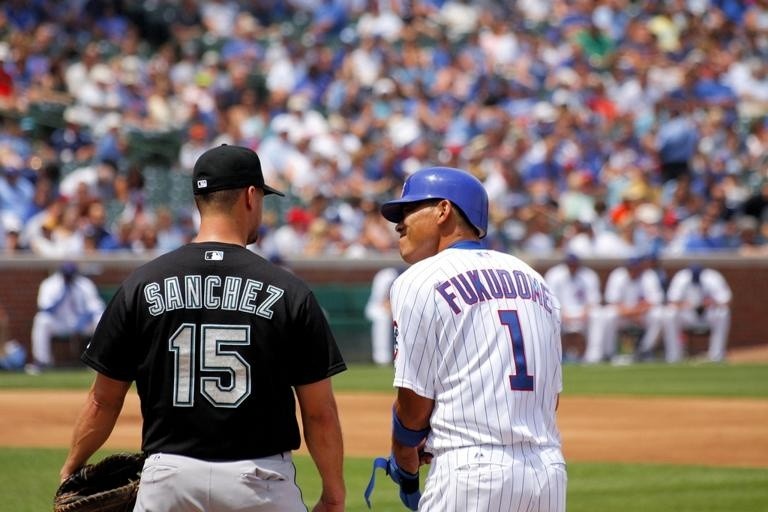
[54,143,347,512]
[0,1,584,373]
[364,168,569,512]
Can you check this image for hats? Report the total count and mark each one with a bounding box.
[194,144,285,197]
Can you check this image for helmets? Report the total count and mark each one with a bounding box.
[382,167,488,238]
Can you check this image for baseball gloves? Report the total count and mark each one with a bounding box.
[54,453,144,511]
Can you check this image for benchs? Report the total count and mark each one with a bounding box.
[53,282,710,366]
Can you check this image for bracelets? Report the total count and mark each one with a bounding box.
[390,412,428,446]
